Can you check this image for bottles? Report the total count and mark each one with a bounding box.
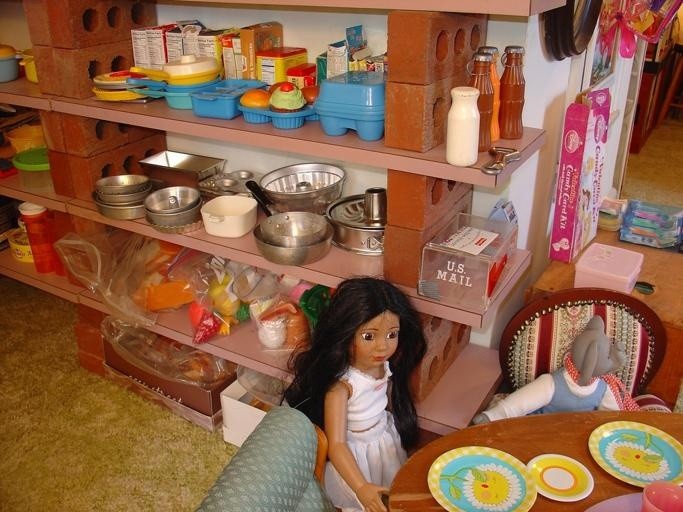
[17,199,60,275]
[445,85,482,167]
[471,46,527,153]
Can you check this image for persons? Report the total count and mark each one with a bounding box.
[281,274,428,512]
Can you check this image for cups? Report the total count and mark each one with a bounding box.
[18,49,41,85]
[640,480,683,512]
[258,303,307,347]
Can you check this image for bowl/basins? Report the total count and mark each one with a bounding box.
[7,124,46,154]
[93,150,387,267]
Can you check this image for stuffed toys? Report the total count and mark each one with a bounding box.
[472,314,640,426]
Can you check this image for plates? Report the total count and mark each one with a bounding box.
[588,419,683,486]
[91,68,156,105]
[581,492,643,512]
[9,147,60,173]
[427,444,536,512]
[525,453,595,502]
